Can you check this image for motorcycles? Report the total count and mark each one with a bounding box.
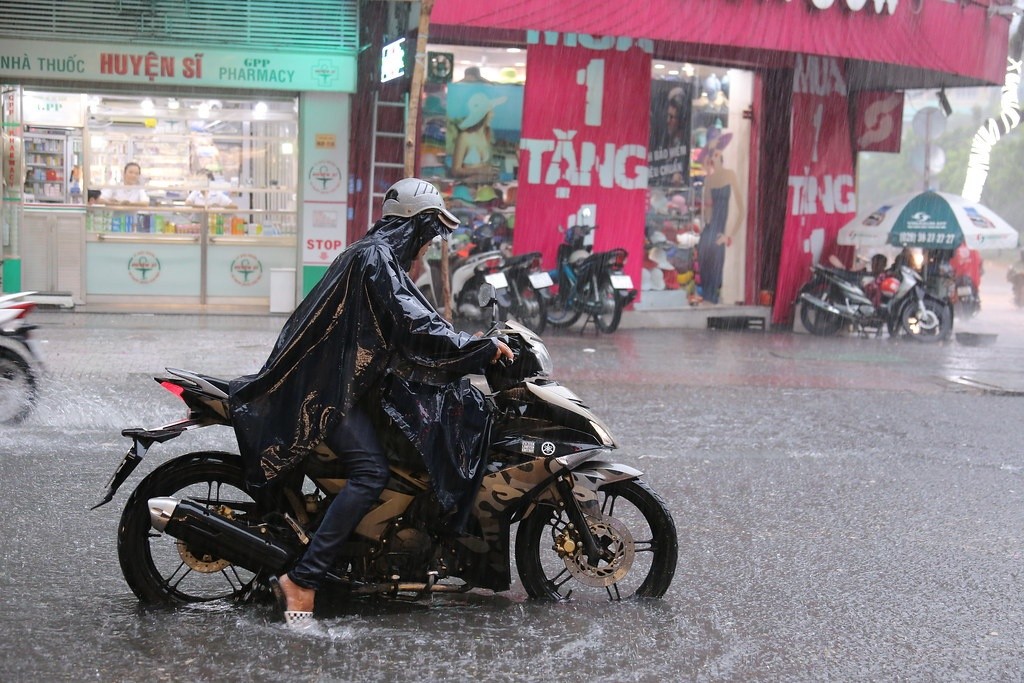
[791,242,951,343]
[85,282,679,617]
[0,290,50,429]
[955,275,981,321]
[544,207,638,338]
[435,220,552,335]
[1008,265,1024,306]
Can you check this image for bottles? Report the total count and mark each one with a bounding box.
[208,213,231,236]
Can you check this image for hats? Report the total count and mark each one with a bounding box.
[640,75,734,301]
[458,92,507,131]
[418,81,517,260]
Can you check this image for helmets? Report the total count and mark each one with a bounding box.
[379,177,462,229]
[879,277,898,297]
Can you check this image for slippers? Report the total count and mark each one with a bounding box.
[267,576,327,639]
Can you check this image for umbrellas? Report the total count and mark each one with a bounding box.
[835,188,1018,286]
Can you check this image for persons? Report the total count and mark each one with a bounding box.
[185,169,238,208]
[99,163,150,206]
[649,88,685,180]
[454,94,507,183]
[278,178,514,625]
[926,242,984,306]
[870,254,886,271]
